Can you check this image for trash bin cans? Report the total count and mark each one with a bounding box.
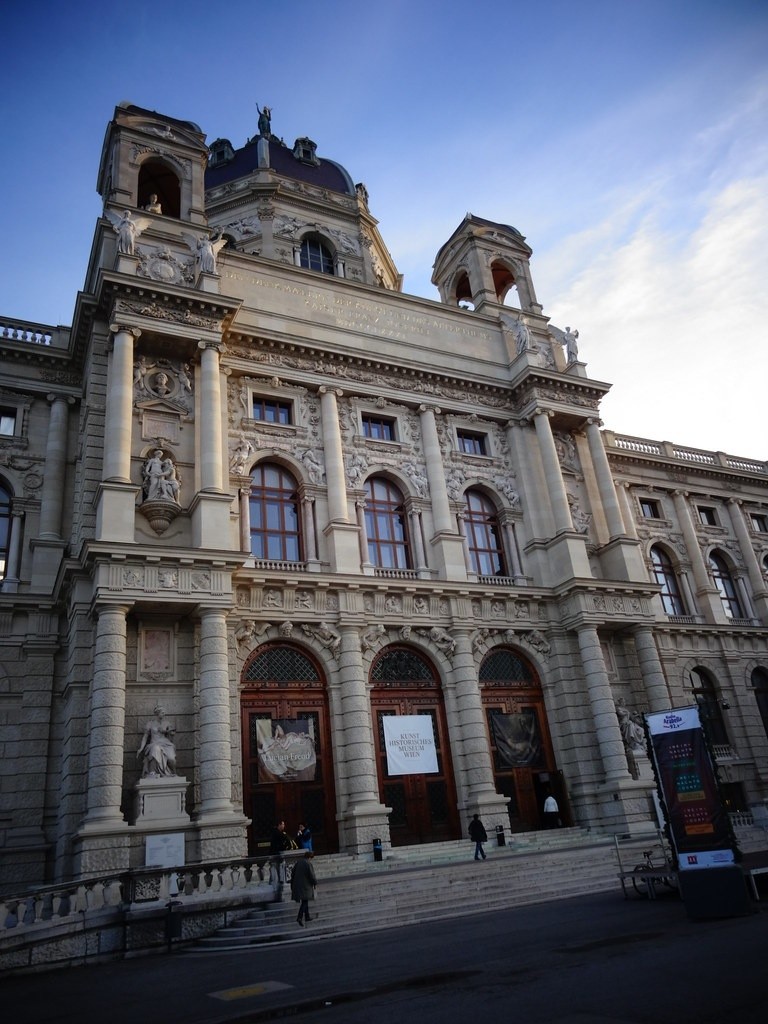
[372,838,383,861]
[165,900,183,938]
[495,825,505,846]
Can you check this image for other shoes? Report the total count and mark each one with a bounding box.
[483,855,486,860]
[474,858,480,860]
[297,918,304,927]
[305,918,313,922]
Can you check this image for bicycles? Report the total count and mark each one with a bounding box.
[632,842,674,893]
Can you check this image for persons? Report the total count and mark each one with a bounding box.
[570,502,592,536]
[564,327,578,364]
[256,103,271,135]
[544,794,559,829]
[228,357,519,506]
[616,697,646,748]
[514,314,533,354]
[113,210,136,255]
[266,819,290,855]
[136,706,177,775]
[468,814,488,860]
[133,355,192,398]
[145,194,162,214]
[197,234,215,273]
[145,450,180,503]
[291,852,316,927]
[295,822,312,851]
